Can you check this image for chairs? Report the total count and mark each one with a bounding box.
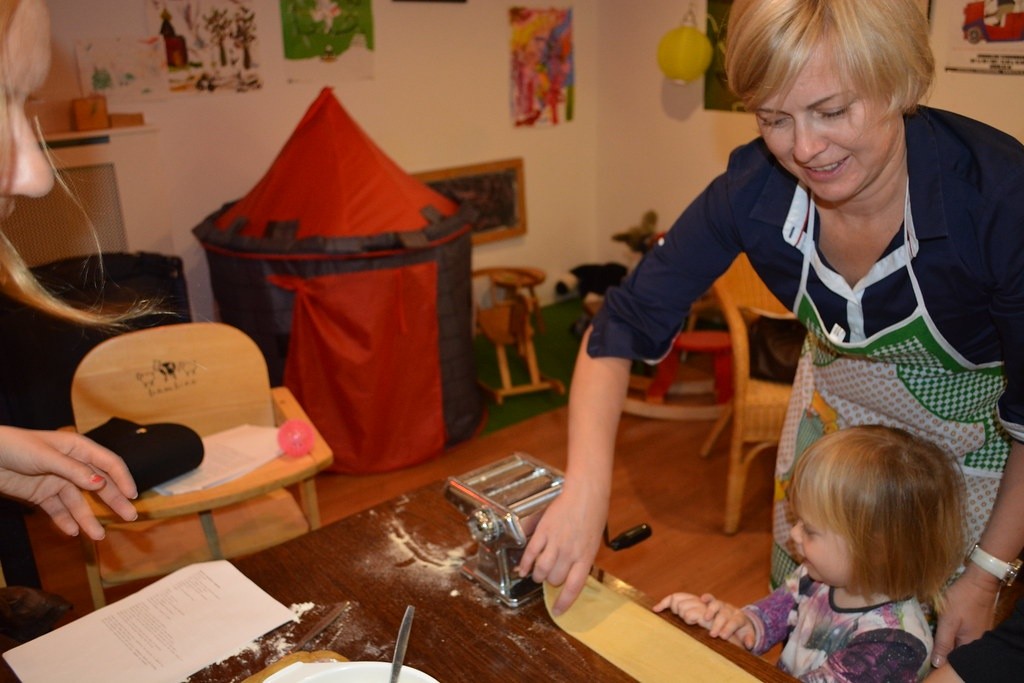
[55,322,335,612]
[711,278,795,536]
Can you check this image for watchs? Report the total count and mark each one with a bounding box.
[969,543,1023,586]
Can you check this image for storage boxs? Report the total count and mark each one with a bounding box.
[71,93,145,132]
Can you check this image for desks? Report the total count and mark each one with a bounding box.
[0,476,807,683]
[698,251,801,457]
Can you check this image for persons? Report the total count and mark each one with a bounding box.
[652,424,964,683]
[0,0,140,683]
[926,596,1024,683]
[520,0,1024,669]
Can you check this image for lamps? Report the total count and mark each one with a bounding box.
[655,0,724,87]
